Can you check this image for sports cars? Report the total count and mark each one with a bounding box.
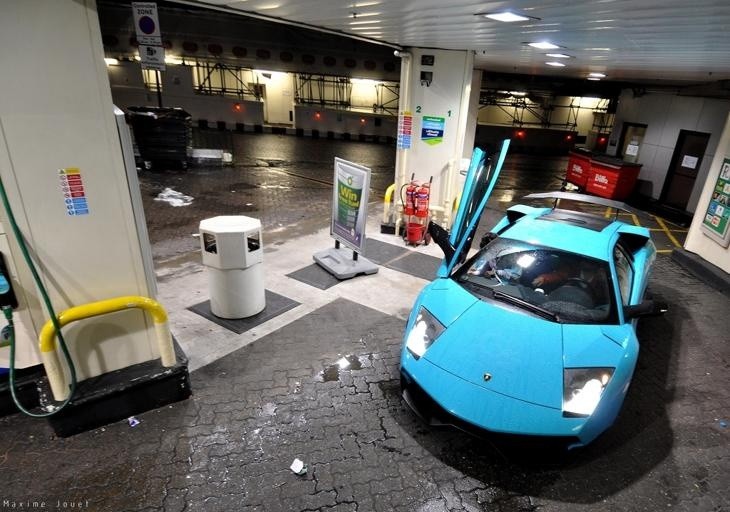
[399,138,668,454]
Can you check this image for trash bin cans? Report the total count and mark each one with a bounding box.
[199,215,266,319]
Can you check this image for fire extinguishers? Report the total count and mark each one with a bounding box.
[400,173,433,218]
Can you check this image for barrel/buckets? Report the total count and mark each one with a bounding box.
[406,222,425,242]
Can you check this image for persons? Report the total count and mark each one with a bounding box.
[532,258,611,311]
[467,231,522,282]
